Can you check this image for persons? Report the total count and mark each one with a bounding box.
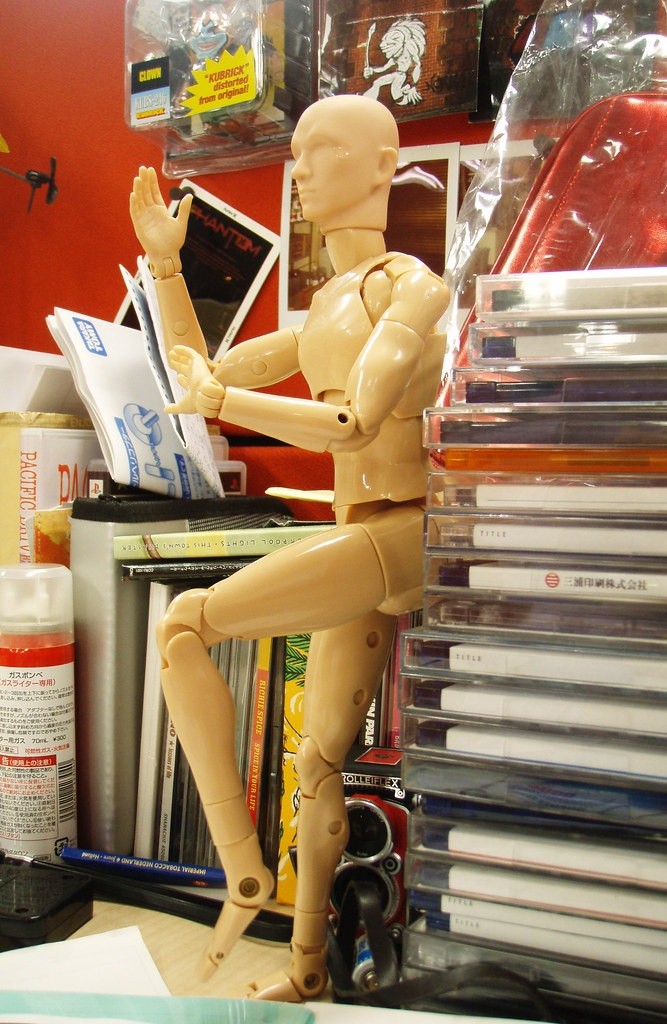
[130,94,452,996]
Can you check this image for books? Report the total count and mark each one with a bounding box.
[114,524,335,888]
